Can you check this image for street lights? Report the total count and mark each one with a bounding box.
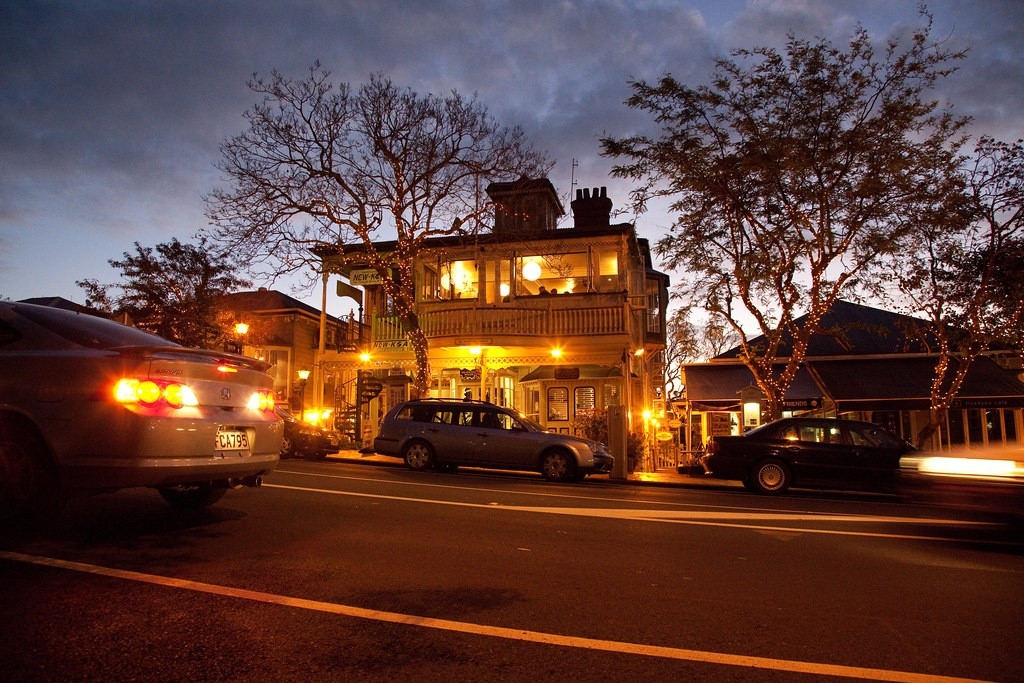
[232,323,249,356]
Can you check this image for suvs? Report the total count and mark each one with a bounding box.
[373,397,616,483]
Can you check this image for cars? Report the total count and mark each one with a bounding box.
[0,300,286,517]
[700,415,935,495]
[268,403,342,459]
